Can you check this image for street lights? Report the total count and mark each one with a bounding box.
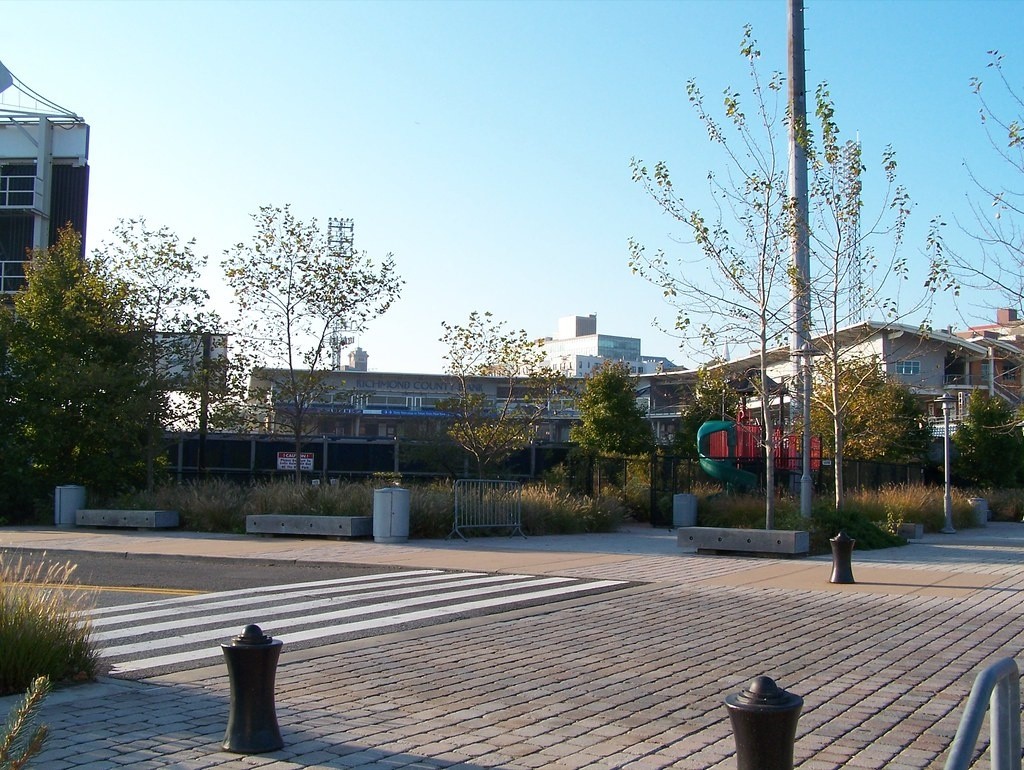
[933,393,957,534]
[789,343,825,518]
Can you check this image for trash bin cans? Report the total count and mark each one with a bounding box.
[373,487,410,543]
[55,485,85,527]
[970,498,987,526]
[673,494,698,527]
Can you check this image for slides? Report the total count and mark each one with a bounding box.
[699,453,758,496]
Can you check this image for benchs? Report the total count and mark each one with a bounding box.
[74,509,181,531]
[246,514,373,541]
[676,526,810,560]
[870,521,924,539]
